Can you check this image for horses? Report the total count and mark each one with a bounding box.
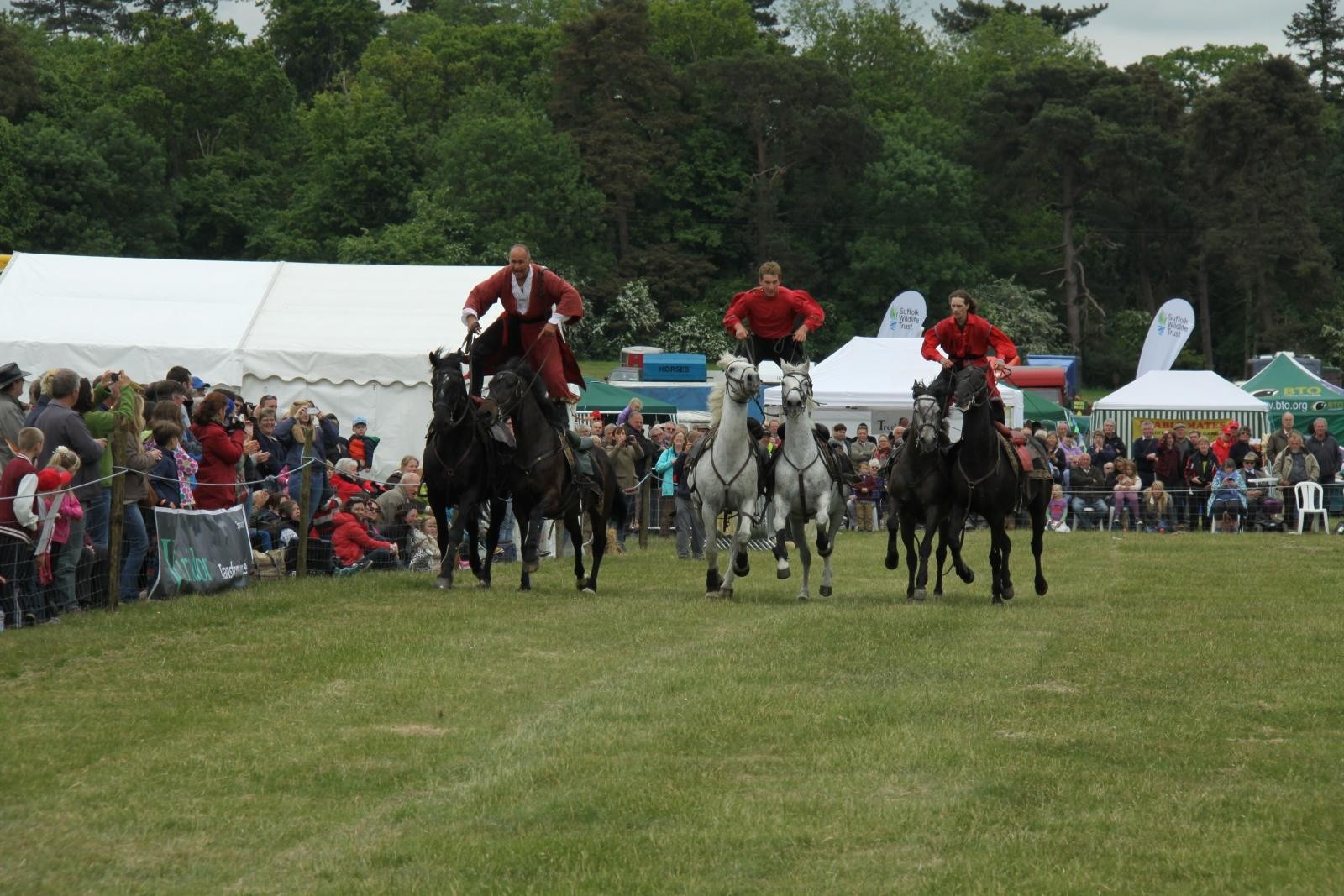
[684,349,770,600]
[421,351,520,591]
[477,357,631,593]
[885,377,953,603]
[762,357,851,601]
[947,360,1051,606]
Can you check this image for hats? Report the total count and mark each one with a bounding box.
[591,410,602,417]
[352,417,367,426]
[1173,423,1185,429]
[191,376,210,389]
[1244,455,1257,461]
[1230,421,1238,428]
[225,398,236,416]
[763,429,772,437]
[37,468,72,489]
[1223,427,1232,434]
[0,363,31,390]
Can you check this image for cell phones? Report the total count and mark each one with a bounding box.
[1228,477,1233,481]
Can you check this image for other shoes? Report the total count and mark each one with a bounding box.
[1135,519,1142,525]
[351,560,374,573]
[335,564,357,577]
[1113,517,1119,523]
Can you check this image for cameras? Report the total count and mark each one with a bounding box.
[306,408,318,415]
[625,437,634,441]
[240,402,256,414]
[111,374,119,381]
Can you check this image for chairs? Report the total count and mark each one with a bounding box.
[1108,505,1141,532]
[846,501,879,531]
[1211,511,1243,534]
[1070,507,1104,531]
[1294,481,1330,536]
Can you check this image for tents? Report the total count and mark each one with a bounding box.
[610,381,771,427]
[1090,369,1271,460]
[763,336,1025,445]
[0,248,560,485]
[1238,351,1344,449]
[998,381,1067,435]
[1069,413,1090,435]
[576,378,677,432]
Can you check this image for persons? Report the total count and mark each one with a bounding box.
[921,289,1018,426]
[721,261,826,367]
[0,361,1344,631]
[461,242,584,401]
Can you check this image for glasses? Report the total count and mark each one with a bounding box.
[266,395,276,400]
[593,417,601,420]
[858,424,868,428]
[666,427,676,431]
[1246,461,1255,464]
[370,506,380,514]
